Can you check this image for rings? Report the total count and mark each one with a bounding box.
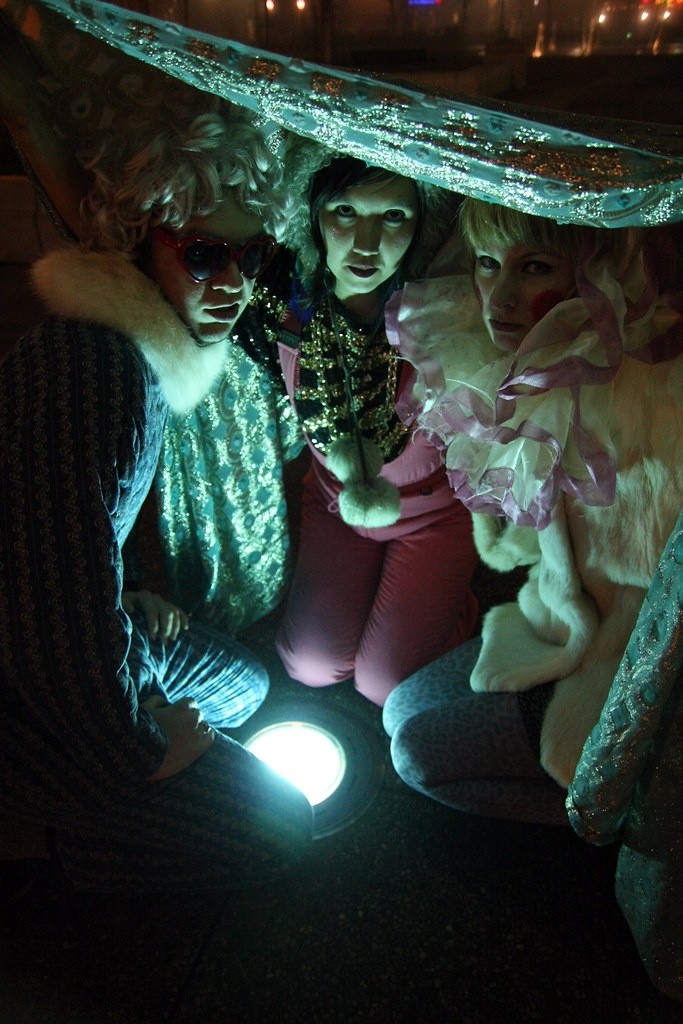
[198,720,212,733]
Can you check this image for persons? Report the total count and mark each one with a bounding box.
[0,96,683,896]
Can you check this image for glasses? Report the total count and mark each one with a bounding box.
[153,229,280,281]
